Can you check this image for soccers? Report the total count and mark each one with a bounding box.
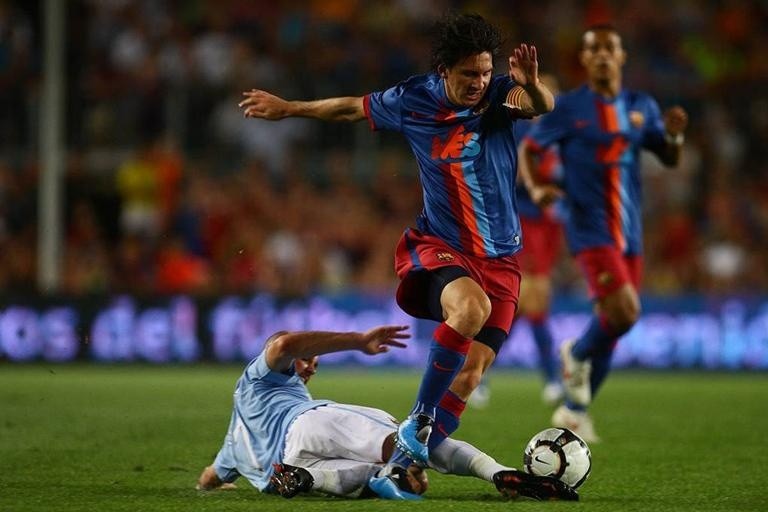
[524,427,591,490]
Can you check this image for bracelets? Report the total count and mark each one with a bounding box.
[663,133,686,147]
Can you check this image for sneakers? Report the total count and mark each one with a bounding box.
[552,404,604,444]
[492,469,581,503]
[368,465,425,501]
[559,336,596,409]
[268,463,313,498]
[394,411,435,466]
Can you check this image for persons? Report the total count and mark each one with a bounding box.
[471,70,565,406]
[515,25,688,445]
[199,324,578,503]
[238,12,555,502]
